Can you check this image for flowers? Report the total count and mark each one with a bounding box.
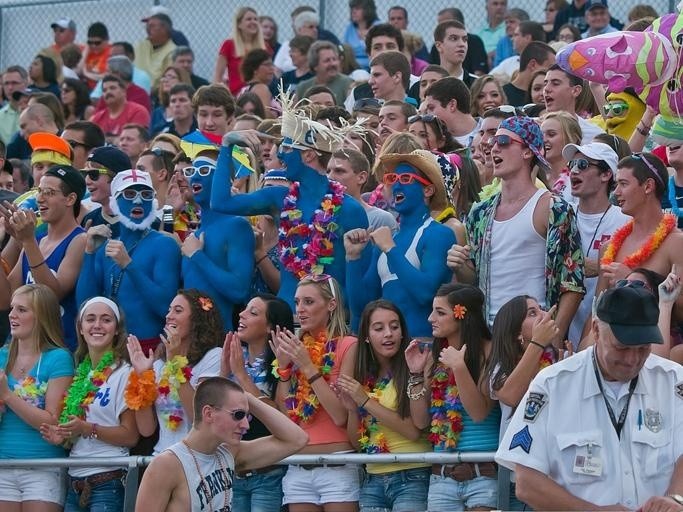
[57,351,113,423]
[200,297,212,310]
[453,302,467,319]
[426,360,463,449]
[277,179,347,280]
[285,336,336,421]
[124,368,159,411]
[12,376,48,410]
[599,211,674,267]
[538,349,552,373]
[157,355,194,431]
[295,331,325,409]
[356,370,395,453]
[230,349,266,436]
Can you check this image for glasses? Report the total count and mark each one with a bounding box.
[183,165,216,177]
[86,39,103,45]
[354,98,378,108]
[79,169,114,180]
[148,145,167,171]
[123,188,156,202]
[497,105,516,117]
[300,273,336,297]
[615,279,651,291]
[65,138,87,148]
[487,134,525,147]
[603,104,629,116]
[383,173,431,186]
[58,87,72,93]
[407,113,442,135]
[211,405,254,423]
[566,158,601,170]
[37,187,63,196]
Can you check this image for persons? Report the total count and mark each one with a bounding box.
[3,2,682,512]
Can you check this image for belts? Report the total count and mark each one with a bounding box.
[294,464,344,470]
[71,470,123,508]
[430,463,497,481]
[237,464,284,478]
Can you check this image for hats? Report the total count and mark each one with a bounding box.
[109,168,156,195]
[583,0,607,11]
[561,142,618,181]
[26,132,73,166]
[280,101,338,154]
[12,88,40,99]
[44,165,86,200]
[379,149,447,211]
[51,15,76,29]
[497,115,551,171]
[596,287,663,345]
[87,146,131,175]
[142,13,171,23]
